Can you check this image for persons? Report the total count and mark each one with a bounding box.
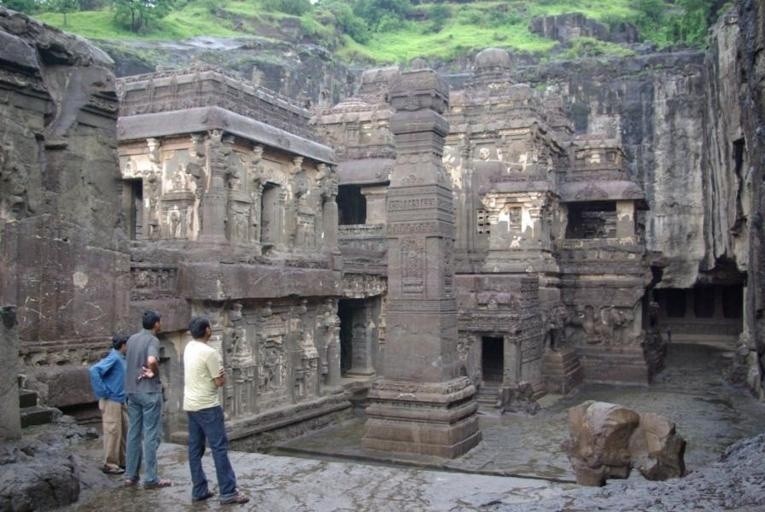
[180,316,249,505]
[122,309,171,490]
[88,333,129,475]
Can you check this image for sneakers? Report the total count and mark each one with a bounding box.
[191,490,214,502]
[219,493,250,506]
[102,462,126,475]
[124,479,172,490]
